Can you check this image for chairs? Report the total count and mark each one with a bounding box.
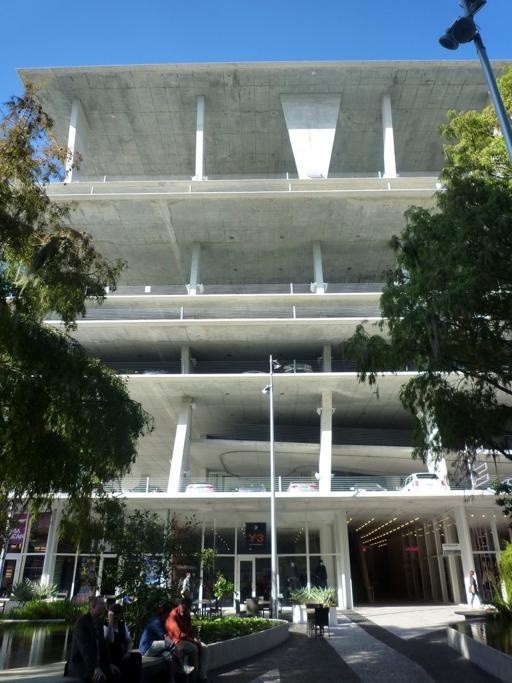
[191,596,331,640]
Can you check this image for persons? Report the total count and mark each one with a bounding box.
[64,596,204,683]
[468,570,482,607]
[180,572,190,603]
[316,560,327,591]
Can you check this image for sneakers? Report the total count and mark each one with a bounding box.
[183,664,195,674]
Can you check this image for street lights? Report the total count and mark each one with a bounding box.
[436,1,512,171]
[261,353,282,621]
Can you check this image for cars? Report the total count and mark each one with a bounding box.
[134,358,313,374]
